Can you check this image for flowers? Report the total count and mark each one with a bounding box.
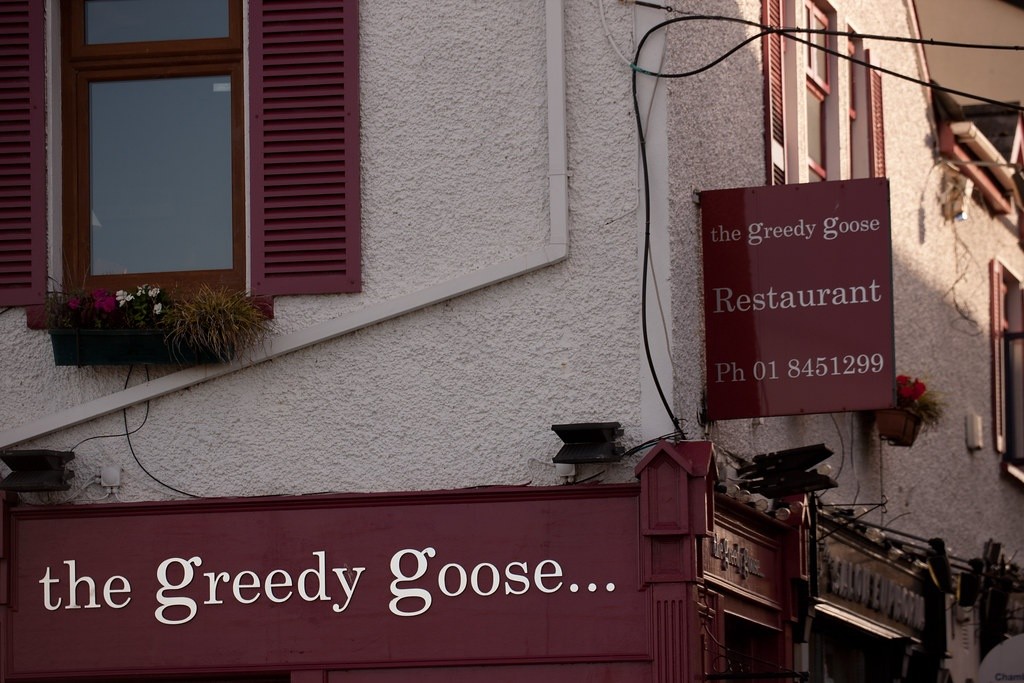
[42,268,172,330]
[896,375,947,427]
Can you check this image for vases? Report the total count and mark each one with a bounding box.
[49,329,171,366]
[875,408,923,448]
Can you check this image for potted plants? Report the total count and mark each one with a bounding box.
[162,282,276,364]
